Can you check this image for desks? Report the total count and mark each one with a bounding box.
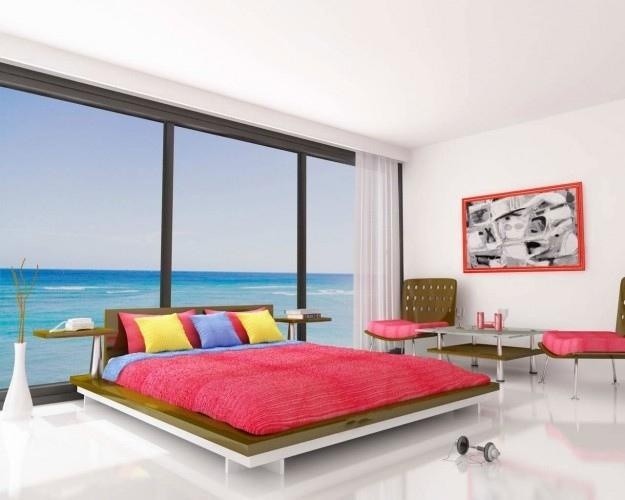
[34,328,116,383]
[275,316,332,340]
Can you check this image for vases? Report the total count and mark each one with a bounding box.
[3,342,34,423]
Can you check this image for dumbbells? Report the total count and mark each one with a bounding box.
[454,435,500,462]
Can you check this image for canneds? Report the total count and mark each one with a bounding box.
[495,313,502,330]
[484,322,495,328]
[477,312,484,329]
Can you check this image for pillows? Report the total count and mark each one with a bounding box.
[117,306,284,356]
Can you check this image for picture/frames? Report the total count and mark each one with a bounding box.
[461,181,586,274]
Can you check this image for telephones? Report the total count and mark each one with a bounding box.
[65,318,94,331]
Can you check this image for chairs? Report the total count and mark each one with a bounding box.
[366,277,457,362]
[537,276,625,402]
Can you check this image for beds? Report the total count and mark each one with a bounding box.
[70,304,482,469]
